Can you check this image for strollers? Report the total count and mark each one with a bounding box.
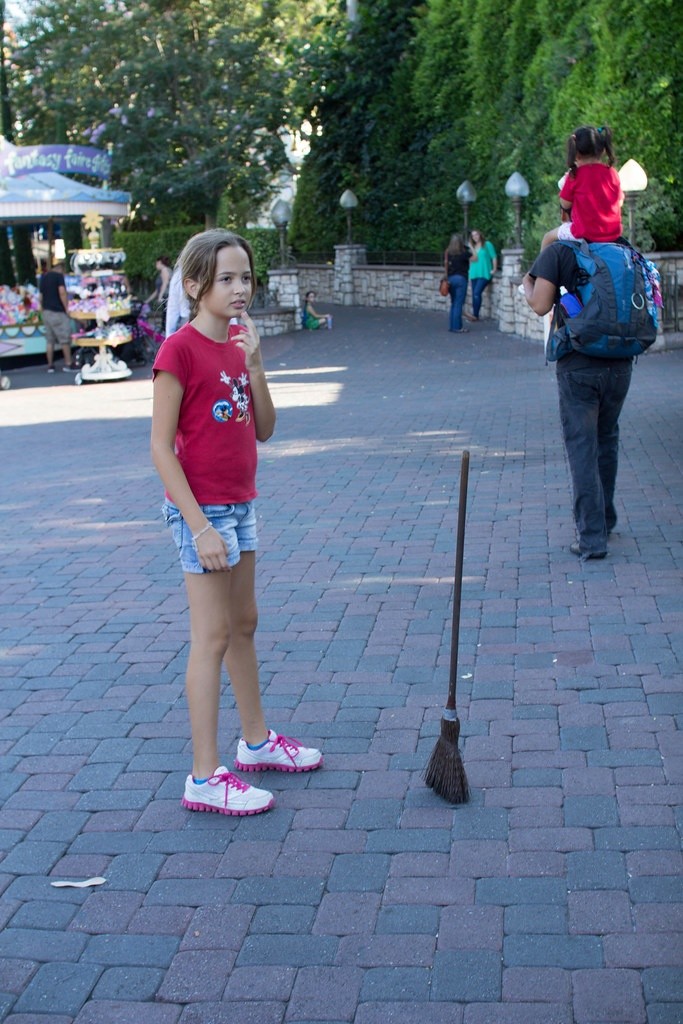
[71,299,147,371]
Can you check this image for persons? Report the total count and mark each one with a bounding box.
[145,256,172,334]
[523,203,648,559]
[539,125,622,249]
[302,291,330,329]
[470,229,497,321]
[36,259,85,373]
[151,227,323,814]
[445,231,477,332]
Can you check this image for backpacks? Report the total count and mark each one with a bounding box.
[555,238,663,360]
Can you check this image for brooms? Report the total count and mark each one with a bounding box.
[417,449,472,806]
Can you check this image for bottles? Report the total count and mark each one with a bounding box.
[327,317,332,329]
[559,286,582,318]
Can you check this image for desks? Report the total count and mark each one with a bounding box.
[0,313,91,358]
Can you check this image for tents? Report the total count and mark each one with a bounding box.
[0,137,132,270]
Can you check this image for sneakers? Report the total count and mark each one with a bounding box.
[181,765,274,816]
[233,729,324,773]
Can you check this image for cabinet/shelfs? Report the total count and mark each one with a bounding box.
[65,247,133,381]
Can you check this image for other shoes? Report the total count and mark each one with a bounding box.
[48,365,55,373]
[449,327,469,332]
[571,542,607,559]
[466,315,479,322]
[62,363,81,372]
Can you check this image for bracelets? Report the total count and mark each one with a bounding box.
[191,522,213,542]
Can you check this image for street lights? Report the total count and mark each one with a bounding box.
[616,157,648,246]
[339,188,359,245]
[455,179,478,246]
[504,170,530,248]
[270,198,291,269]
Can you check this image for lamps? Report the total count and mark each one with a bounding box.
[456,179,477,204]
[339,190,359,208]
[619,158,647,191]
[505,171,530,197]
[270,199,291,225]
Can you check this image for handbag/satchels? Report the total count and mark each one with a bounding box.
[439,279,450,296]
